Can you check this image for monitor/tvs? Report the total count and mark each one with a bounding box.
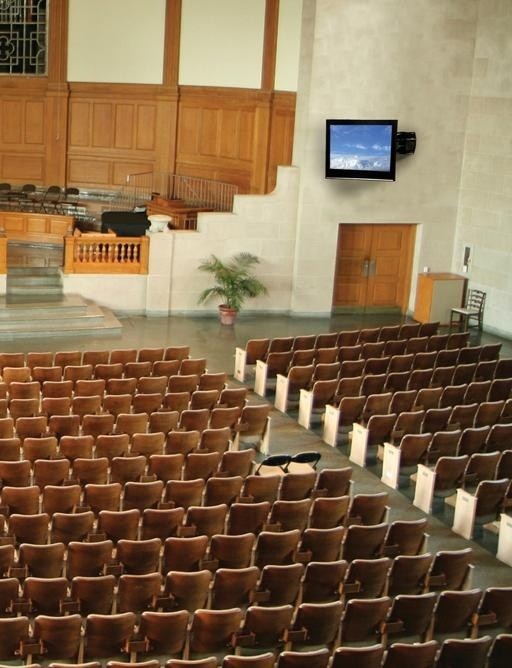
[325,118,398,182]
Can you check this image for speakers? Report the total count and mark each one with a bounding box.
[396,131,417,155]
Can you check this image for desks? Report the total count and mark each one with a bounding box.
[146,199,214,231]
[413,273,468,326]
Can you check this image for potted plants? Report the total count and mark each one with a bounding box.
[195,252,269,326]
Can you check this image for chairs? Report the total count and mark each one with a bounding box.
[449,288,485,334]
[0,179,80,216]
[74,228,116,237]
